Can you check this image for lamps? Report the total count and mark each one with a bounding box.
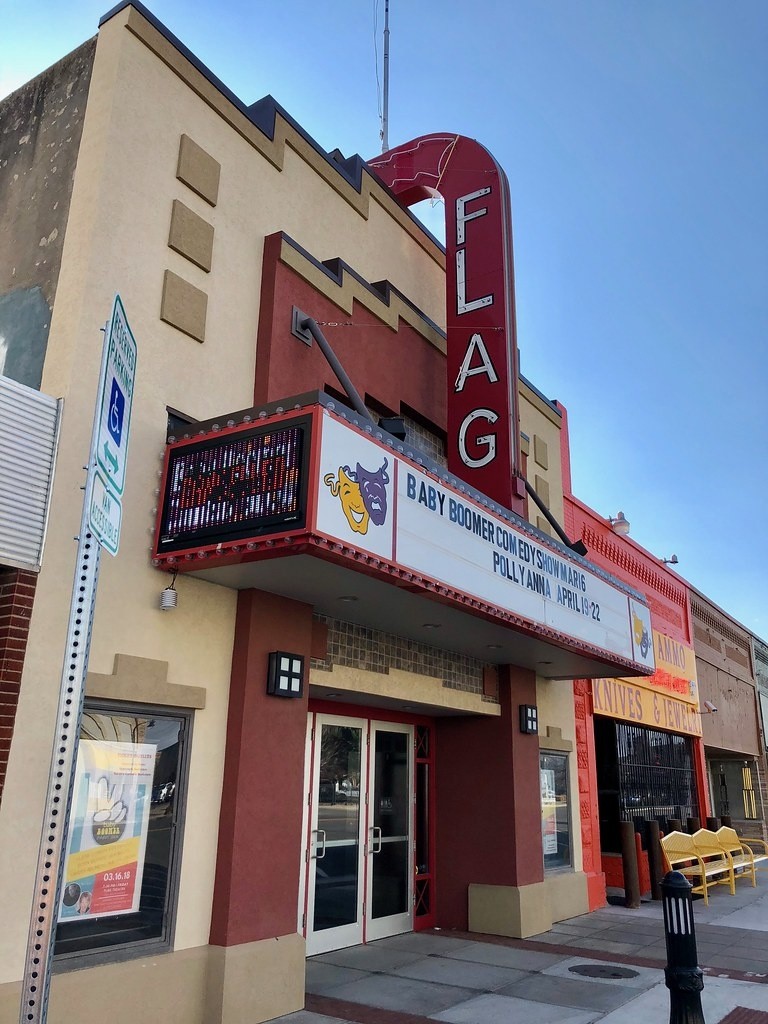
[159,588,177,610]
[603,511,631,536]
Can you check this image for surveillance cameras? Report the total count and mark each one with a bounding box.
[705,701,718,713]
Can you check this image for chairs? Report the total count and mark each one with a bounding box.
[659,826,768,906]
